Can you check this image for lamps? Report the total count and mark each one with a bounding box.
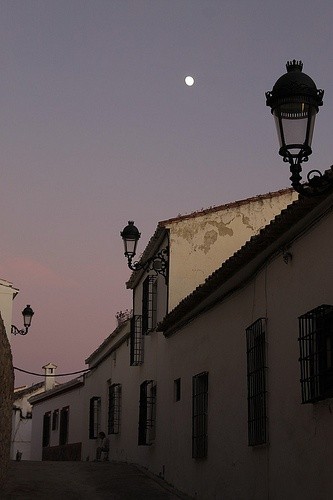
[120,219,168,285]
[10,305,35,336]
[263,59,326,193]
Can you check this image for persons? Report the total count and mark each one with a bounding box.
[94,431,109,461]
